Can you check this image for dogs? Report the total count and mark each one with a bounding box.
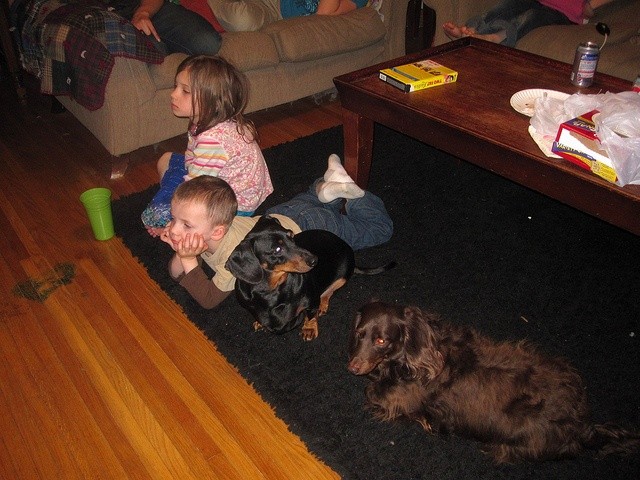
[224,214,391,342]
[345,301,639,470]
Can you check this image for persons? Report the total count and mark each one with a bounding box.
[176,0,372,33]
[160,154,393,311]
[97,0,222,57]
[142,55,275,239]
[441,0,609,49]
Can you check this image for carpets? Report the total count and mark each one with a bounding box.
[105,120,640,477]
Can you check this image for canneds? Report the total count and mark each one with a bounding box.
[570,41,600,88]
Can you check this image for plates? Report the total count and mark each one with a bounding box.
[510,88,578,117]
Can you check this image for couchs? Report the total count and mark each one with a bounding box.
[14,0,408,181]
[424,2,640,84]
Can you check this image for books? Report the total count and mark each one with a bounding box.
[378,59,458,93]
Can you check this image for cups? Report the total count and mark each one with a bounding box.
[79,188,114,241]
